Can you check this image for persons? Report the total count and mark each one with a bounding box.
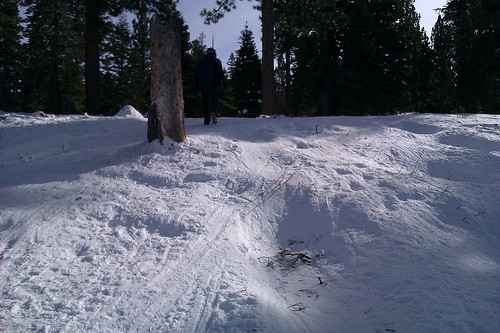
[195,47,227,125]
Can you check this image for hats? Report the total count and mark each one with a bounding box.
[207,48,215,53]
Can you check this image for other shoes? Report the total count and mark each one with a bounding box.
[212,117,217,123]
[204,119,211,125]
